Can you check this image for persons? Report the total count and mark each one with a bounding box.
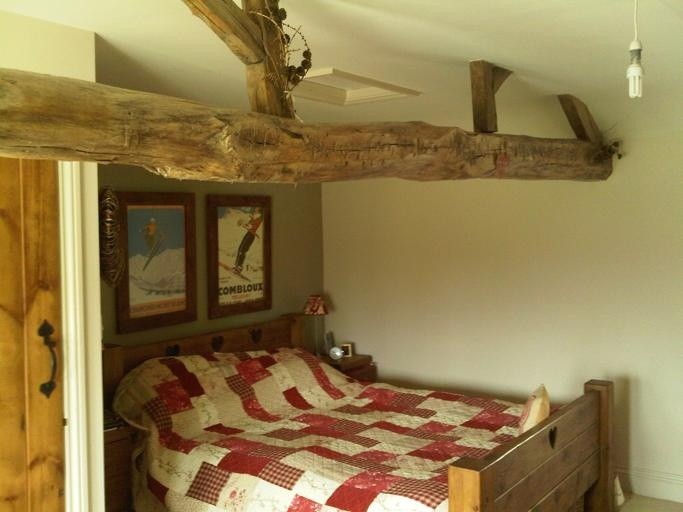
[232,213,264,274]
[139,218,166,248]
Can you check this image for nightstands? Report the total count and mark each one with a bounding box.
[321,351,377,383]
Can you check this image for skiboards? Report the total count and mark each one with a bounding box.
[143,239,163,271]
[219,262,251,283]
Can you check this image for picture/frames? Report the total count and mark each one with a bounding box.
[113,189,274,335]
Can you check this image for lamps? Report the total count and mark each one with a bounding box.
[304,294,328,355]
[627,1,644,100]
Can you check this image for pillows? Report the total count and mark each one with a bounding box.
[113,343,348,438]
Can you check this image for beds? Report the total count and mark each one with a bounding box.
[102,317,615,511]
